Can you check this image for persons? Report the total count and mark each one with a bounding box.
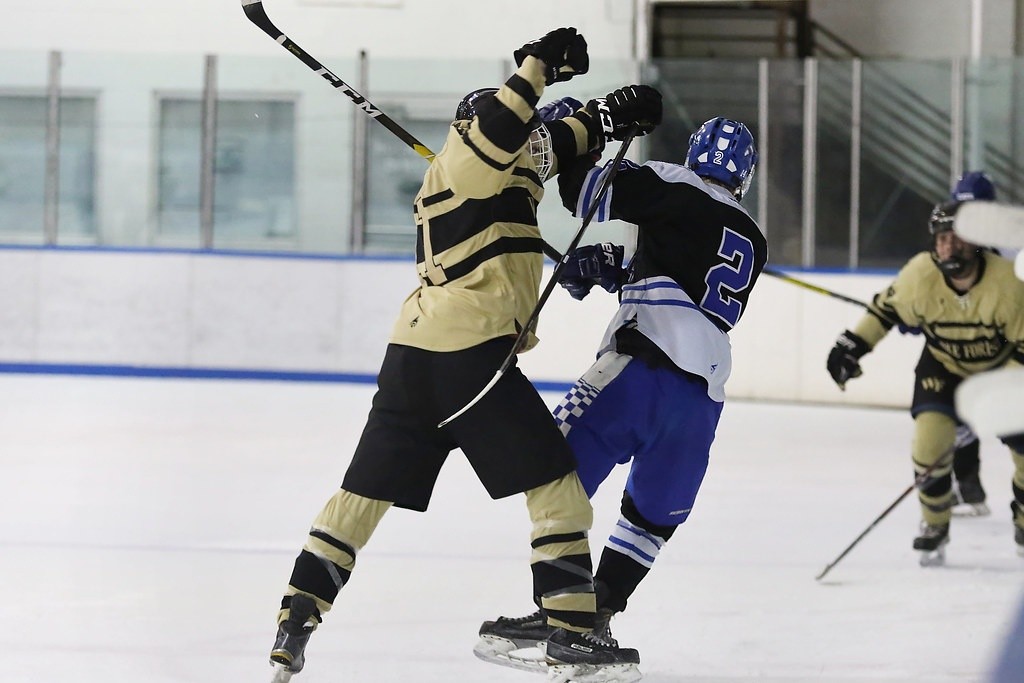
[473,96,767,672]
[825,200,1024,569]
[952,172,998,517]
[269,25,661,683]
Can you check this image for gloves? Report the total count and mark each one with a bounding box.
[587,84,663,147]
[556,241,625,301]
[538,95,584,124]
[513,27,589,87]
[826,330,872,391]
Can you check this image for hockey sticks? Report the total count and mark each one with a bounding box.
[435,127,642,430]
[242,1,566,262]
[813,430,972,583]
[761,266,869,309]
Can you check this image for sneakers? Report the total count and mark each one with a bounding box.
[544,624,642,683]
[949,485,990,516]
[474,592,616,671]
[1013,526,1024,556]
[268,593,318,683]
[912,520,950,565]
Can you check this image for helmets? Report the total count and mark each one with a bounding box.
[926,200,982,277]
[684,116,759,195]
[953,171,998,201]
[456,87,552,184]
